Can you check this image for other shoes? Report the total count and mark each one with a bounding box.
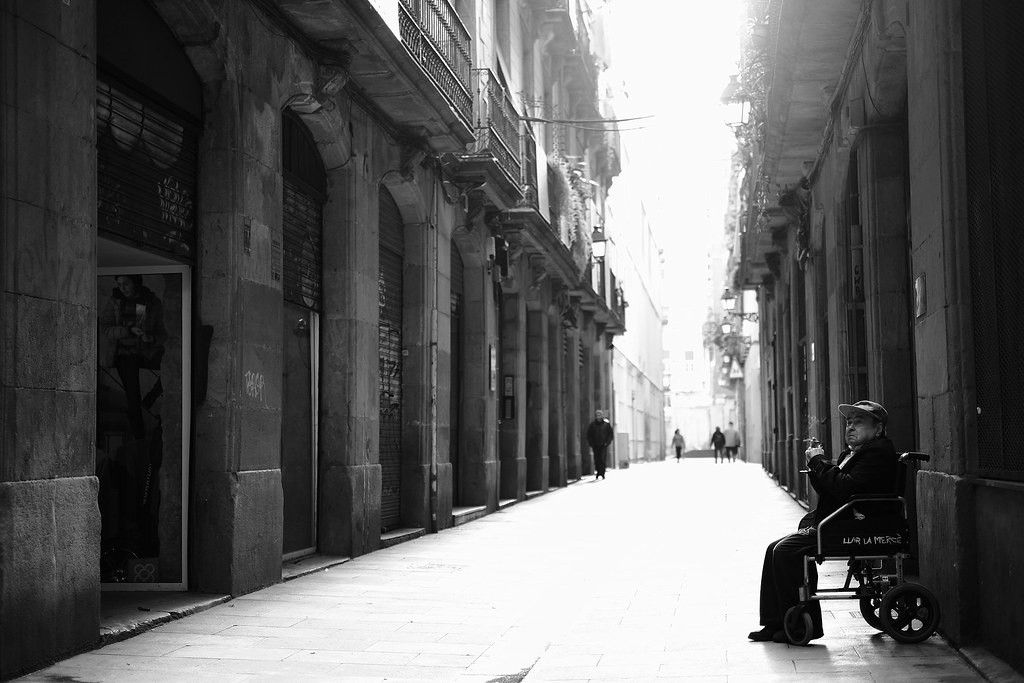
[748,622,824,644]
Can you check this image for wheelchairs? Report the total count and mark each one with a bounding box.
[783,450,939,646]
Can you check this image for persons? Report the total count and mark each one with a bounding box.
[586,410,615,479]
[710,427,725,463]
[670,429,685,463]
[723,421,739,463]
[747,400,900,643]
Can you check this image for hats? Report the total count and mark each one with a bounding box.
[839,401,889,427]
